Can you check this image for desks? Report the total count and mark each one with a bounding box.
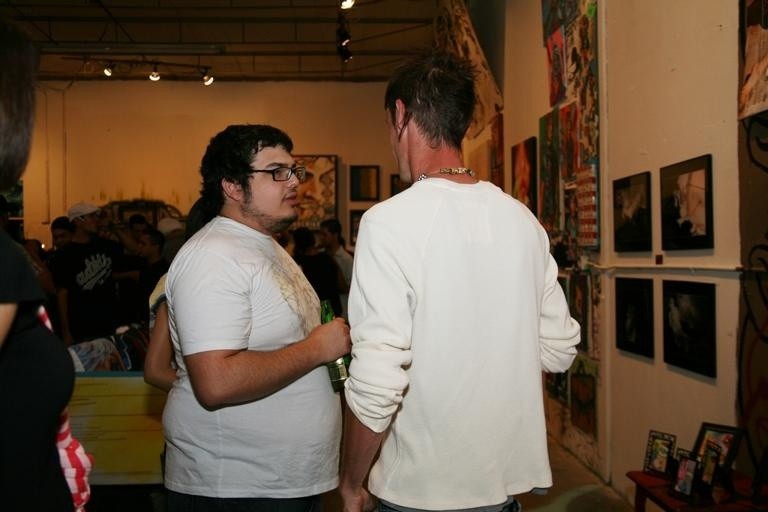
[626,471,767,512]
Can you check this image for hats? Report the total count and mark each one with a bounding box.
[157,218,185,236]
[68,202,102,224]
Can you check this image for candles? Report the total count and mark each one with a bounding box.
[390,174,410,196]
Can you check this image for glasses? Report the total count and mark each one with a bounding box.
[250,166,307,182]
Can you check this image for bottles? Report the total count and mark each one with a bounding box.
[318,299,354,396]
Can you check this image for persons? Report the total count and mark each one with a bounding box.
[163,126,352,512]
[48,206,354,371]
[341,45,580,512]
[0,31,76,512]
[145,198,211,392]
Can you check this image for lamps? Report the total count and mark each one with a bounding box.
[64,57,216,87]
[335,1,354,64]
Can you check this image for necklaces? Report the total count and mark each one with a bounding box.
[415,168,477,177]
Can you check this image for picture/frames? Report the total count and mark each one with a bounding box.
[290,154,338,233]
[349,210,365,246]
[350,166,379,201]
[613,171,652,250]
[663,279,716,378]
[643,422,743,504]
[660,155,714,250]
[615,277,654,358]
